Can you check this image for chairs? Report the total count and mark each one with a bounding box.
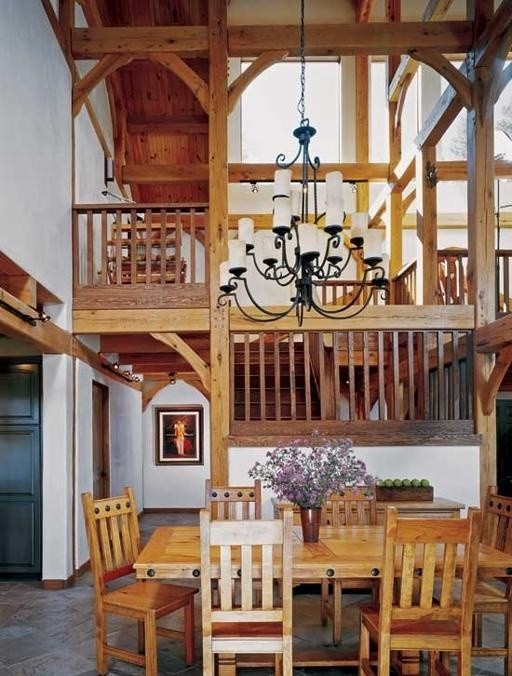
[81,486,198,676]
[319,490,389,647]
[433,484,512,646]
[199,508,294,676]
[205,479,261,607]
[359,505,481,676]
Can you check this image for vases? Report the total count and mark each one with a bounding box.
[300,508,321,544]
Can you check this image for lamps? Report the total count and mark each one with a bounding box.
[113,362,119,370]
[124,371,130,376]
[134,376,140,383]
[169,372,176,383]
[218,1,391,326]
[38,311,51,322]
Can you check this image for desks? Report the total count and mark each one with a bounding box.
[271,498,465,527]
[133,526,512,648]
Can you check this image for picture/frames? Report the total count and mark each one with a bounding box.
[155,407,204,466]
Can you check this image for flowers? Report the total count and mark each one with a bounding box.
[247,429,375,507]
